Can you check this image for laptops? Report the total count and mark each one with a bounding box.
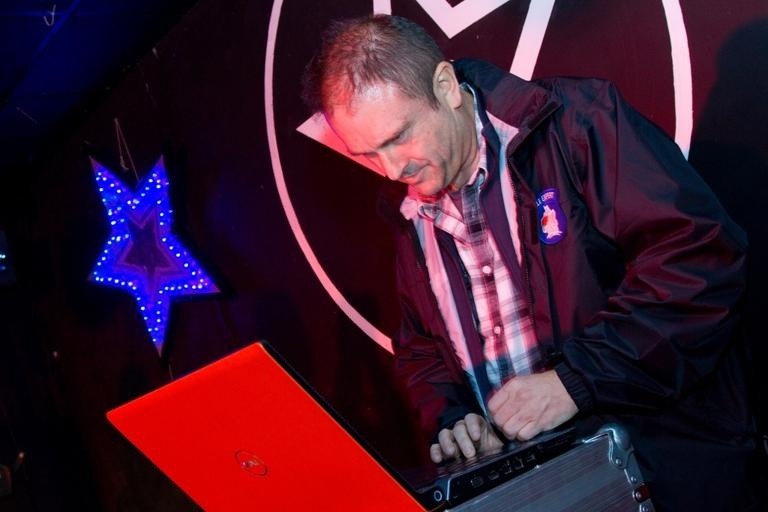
[100,338,576,511]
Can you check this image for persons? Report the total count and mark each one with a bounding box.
[302,12,750,511]
